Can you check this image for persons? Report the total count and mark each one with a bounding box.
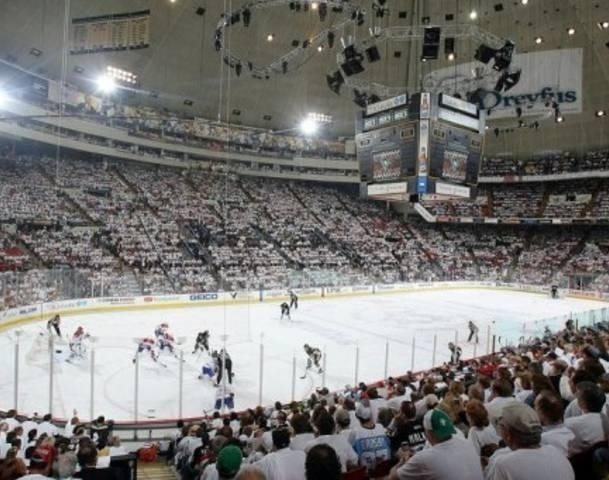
[0,409,126,480]
[26,330,44,363]
[1,148,608,316]
[166,318,609,480]
[133,322,181,363]
[47,313,91,364]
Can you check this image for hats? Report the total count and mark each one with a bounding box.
[424,407,457,439]
[30,446,57,463]
[497,400,543,433]
[217,445,243,474]
